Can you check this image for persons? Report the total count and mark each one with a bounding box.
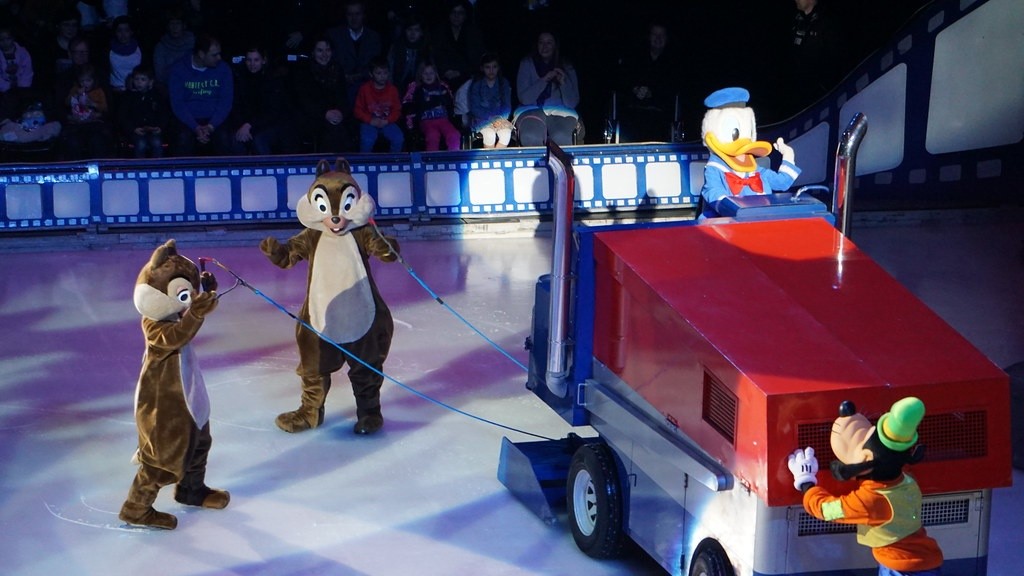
[0,0,687,162]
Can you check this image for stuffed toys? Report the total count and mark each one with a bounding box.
[787,394,944,575]
[259,159,400,433]
[118,238,230,532]
[695,87,802,222]
